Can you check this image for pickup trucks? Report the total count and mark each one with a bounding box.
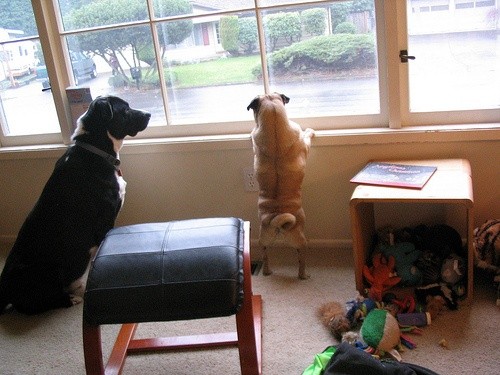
[35,49,98,88]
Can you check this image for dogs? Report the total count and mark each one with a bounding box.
[0,94,151,316]
[246,92,315,281]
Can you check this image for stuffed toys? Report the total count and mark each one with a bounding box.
[318,226,467,360]
[473,218,500,306]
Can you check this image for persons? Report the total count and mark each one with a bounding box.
[108,53,119,76]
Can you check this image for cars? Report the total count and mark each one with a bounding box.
[6,54,39,78]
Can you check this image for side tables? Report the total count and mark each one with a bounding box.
[350,159,476,307]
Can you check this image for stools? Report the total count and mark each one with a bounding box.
[81,217,263,375]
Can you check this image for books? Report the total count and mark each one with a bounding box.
[349,160,437,189]
